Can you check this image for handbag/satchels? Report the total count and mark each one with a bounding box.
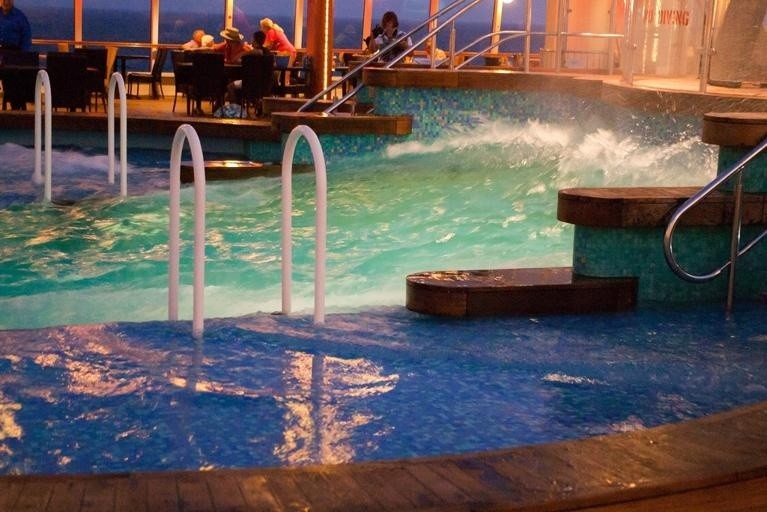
[214,104,247,118]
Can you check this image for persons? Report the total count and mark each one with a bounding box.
[227,31,271,103]
[201,34,214,48]
[260,17,297,86]
[184,29,205,48]
[0,1,33,110]
[219,27,251,65]
[367,11,413,64]
[424,37,447,60]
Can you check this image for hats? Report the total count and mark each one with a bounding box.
[220,27,244,41]
